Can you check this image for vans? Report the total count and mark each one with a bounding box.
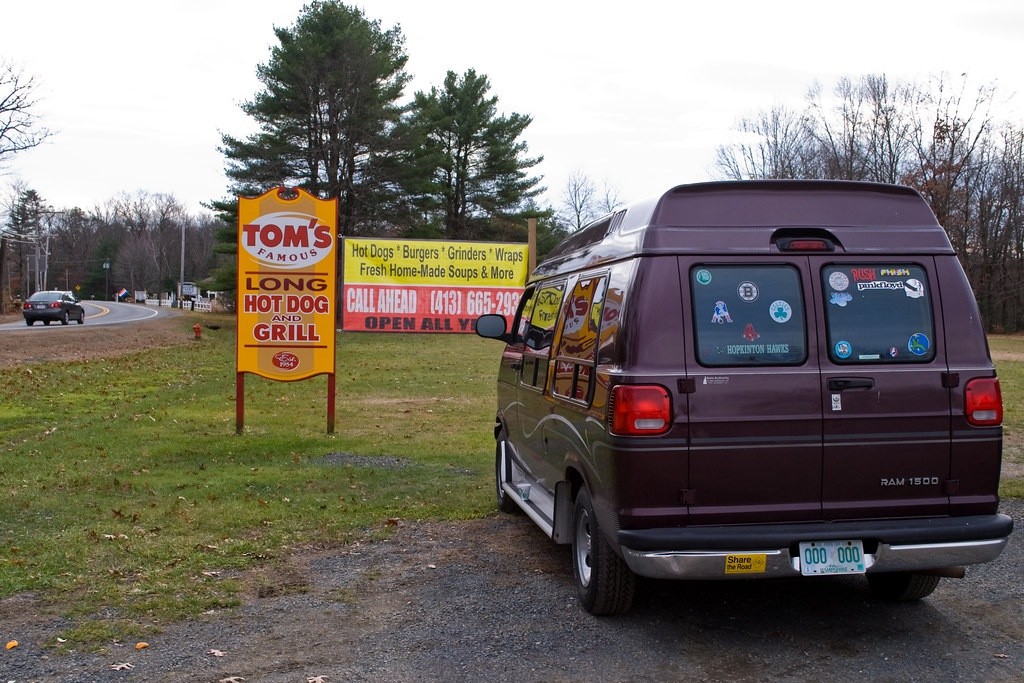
[473,178,1014,617]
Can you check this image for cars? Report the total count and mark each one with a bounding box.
[21,290,86,326]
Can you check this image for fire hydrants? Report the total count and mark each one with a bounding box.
[193,323,201,339]
